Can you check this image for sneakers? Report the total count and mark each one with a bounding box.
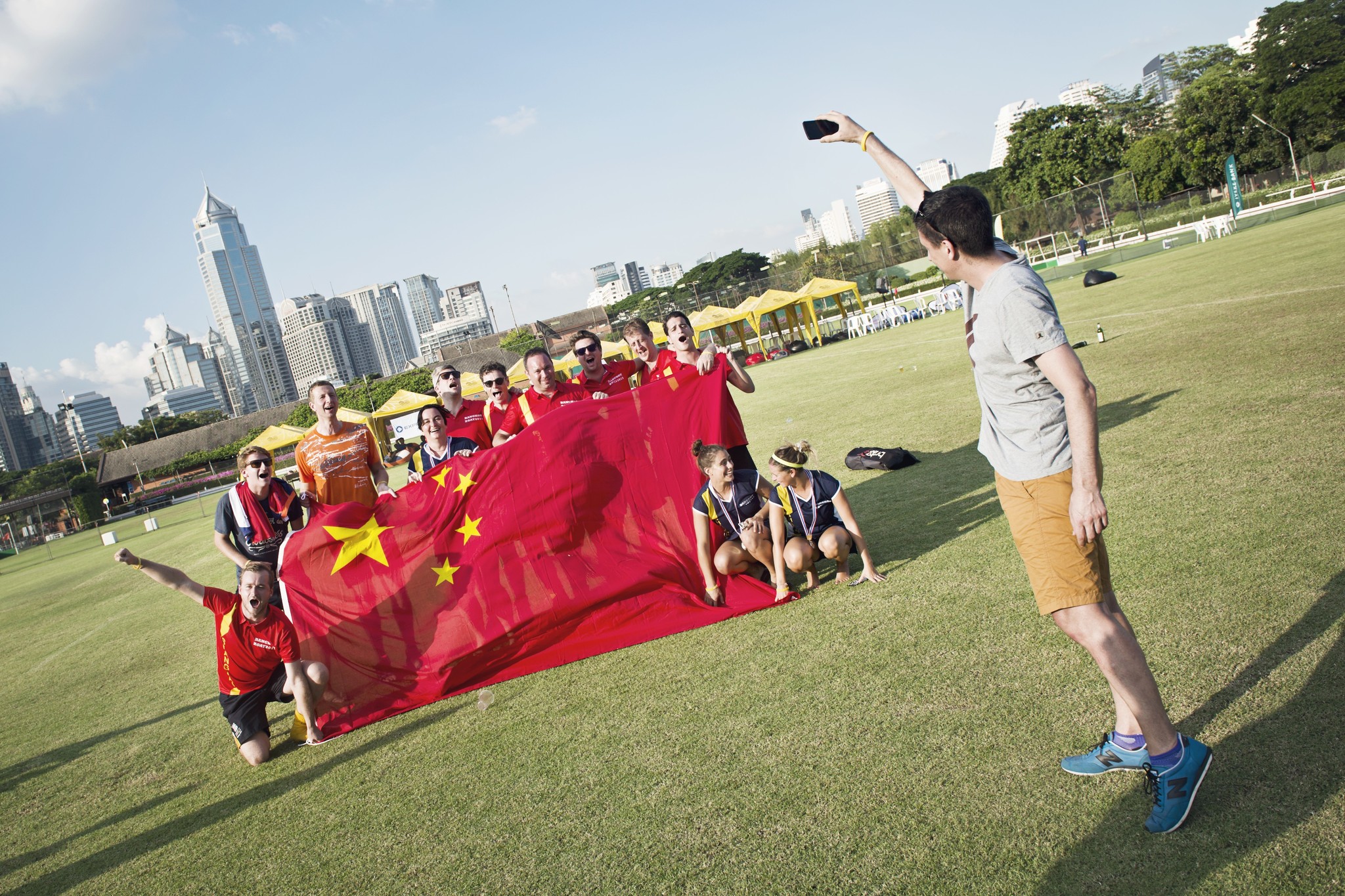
[1060,731,1155,776]
[1142,733,1213,835]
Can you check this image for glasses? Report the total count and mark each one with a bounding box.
[436,371,461,385]
[575,343,599,356]
[914,201,957,248]
[483,376,507,387]
[244,458,273,468]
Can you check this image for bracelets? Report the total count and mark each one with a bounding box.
[375,480,389,489]
[705,584,718,591]
[857,130,875,151]
[242,560,252,568]
[131,555,142,570]
[774,587,790,593]
[699,352,715,356]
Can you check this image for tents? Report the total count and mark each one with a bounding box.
[185,268,868,472]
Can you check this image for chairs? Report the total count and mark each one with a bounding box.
[1193,215,1233,243]
[747,289,962,354]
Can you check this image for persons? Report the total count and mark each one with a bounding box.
[680,438,775,607]
[621,312,715,542]
[429,362,536,635]
[216,445,304,613]
[114,546,334,766]
[1075,234,1089,257]
[490,345,612,599]
[292,379,432,683]
[646,312,764,476]
[474,361,584,611]
[809,106,1218,839]
[564,330,699,569]
[406,403,511,652]
[766,438,887,603]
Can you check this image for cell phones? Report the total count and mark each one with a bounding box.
[803,118,840,142]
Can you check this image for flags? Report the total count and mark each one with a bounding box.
[2,533,9,542]
[894,288,898,295]
[1310,175,1316,191]
[279,354,790,748]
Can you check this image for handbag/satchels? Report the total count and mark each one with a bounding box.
[845,447,921,471]
